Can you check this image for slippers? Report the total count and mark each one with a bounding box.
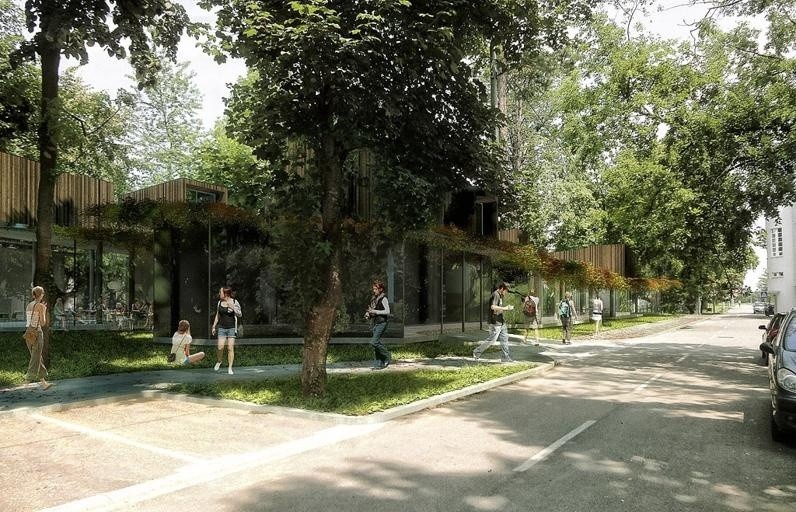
[43,383,53,390]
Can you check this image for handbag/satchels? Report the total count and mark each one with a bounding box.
[167,354,177,362]
[23,326,39,345]
[238,326,244,337]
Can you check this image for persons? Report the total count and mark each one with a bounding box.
[520,289,540,346]
[471,281,515,363]
[559,291,578,345]
[21,285,55,392]
[53,291,155,331]
[170,320,205,366]
[362,279,390,369]
[211,285,243,375]
[591,294,604,335]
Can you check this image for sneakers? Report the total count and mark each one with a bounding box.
[372,360,389,370]
[228,369,233,375]
[215,361,221,370]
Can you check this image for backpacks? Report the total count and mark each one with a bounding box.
[559,298,572,318]
[523,296,536,316]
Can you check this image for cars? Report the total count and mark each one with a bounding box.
[753,302,796,443]
